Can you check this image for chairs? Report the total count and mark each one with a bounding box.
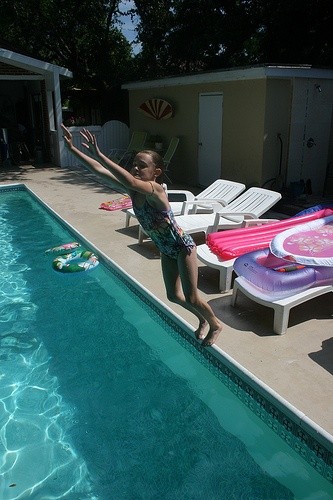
[103,132,179,192]
[127,178,333,336]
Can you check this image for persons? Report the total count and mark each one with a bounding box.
[60,123,222,346]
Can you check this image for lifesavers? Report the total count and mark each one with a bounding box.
[52,250,101,274]
[44,241,81,258]
